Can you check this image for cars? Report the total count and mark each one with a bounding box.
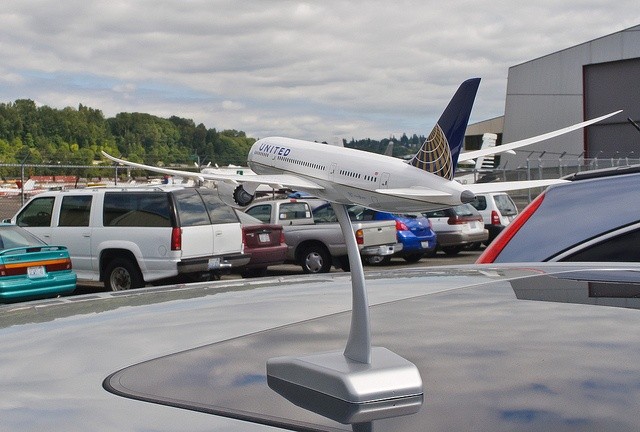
[234,209,288,277]
[0,221,76,303]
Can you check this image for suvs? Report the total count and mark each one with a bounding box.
[473,163,639,264]
[312,203,436,265]
[462,191,518,245]
[428,203,488,254]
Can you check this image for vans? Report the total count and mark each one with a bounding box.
[2,185,250,290]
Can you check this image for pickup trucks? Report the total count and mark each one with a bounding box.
[243,198,402,273]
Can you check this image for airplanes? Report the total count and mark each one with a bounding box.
[101,77,623,362]
